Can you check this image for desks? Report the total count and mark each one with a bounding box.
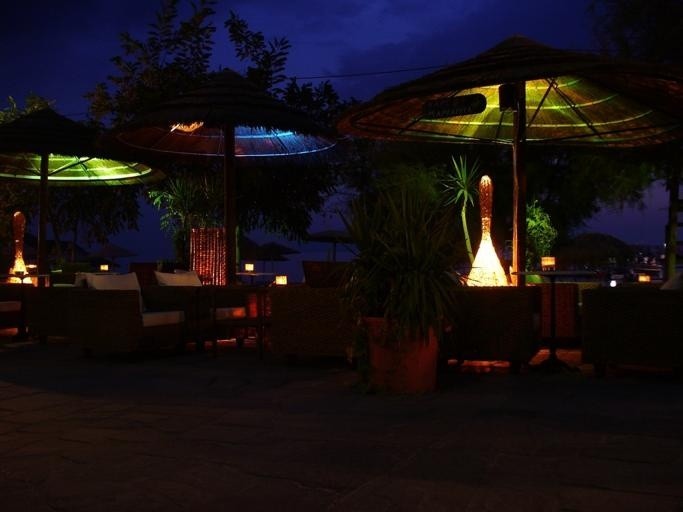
[509,270,594,386]
[161,284,266,367]
[235,272,275,284]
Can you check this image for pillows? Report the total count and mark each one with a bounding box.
[81,272,144,314]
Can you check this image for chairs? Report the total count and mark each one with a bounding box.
[55,271,146,364]
[0,274,52,358]
[583,273,683,400]
[445,285,541,403]
[155,269,202,287]
[264,256,375,373]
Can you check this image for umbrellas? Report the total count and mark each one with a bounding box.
[236,222,360,283]
[116,67,340,287]
[0,105,168,346]
[335,33,682,286]
[105,242,136,265]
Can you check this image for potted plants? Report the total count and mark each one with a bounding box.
[361,172,466,396]
